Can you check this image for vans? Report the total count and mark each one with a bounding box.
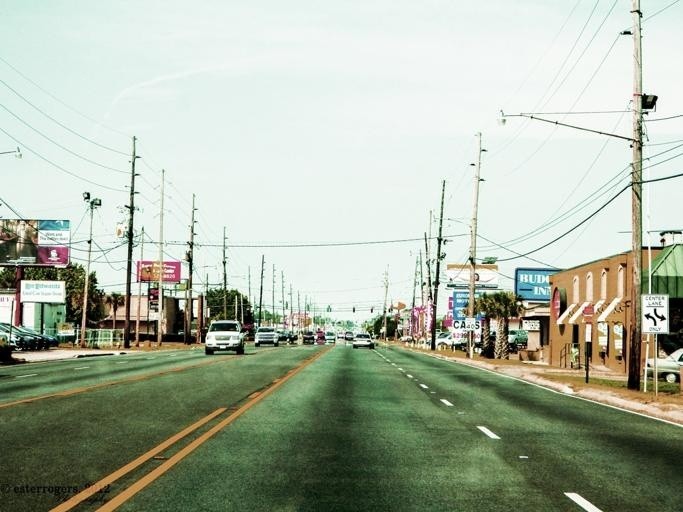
[426,332,461,349]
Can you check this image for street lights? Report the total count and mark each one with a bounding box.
[71,190,101,349]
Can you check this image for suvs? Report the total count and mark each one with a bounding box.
[204,319,247,354]
[253,327,280,347]
[344,332,353,340]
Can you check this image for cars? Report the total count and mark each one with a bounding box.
[352,333,374,349]
[278,327,335,344]
[398,328,442,344]
[337,331,344,339]
[488,331,496,338]
[0,323,59,351]
[646,347,682,384]
[504,330,528,347]
